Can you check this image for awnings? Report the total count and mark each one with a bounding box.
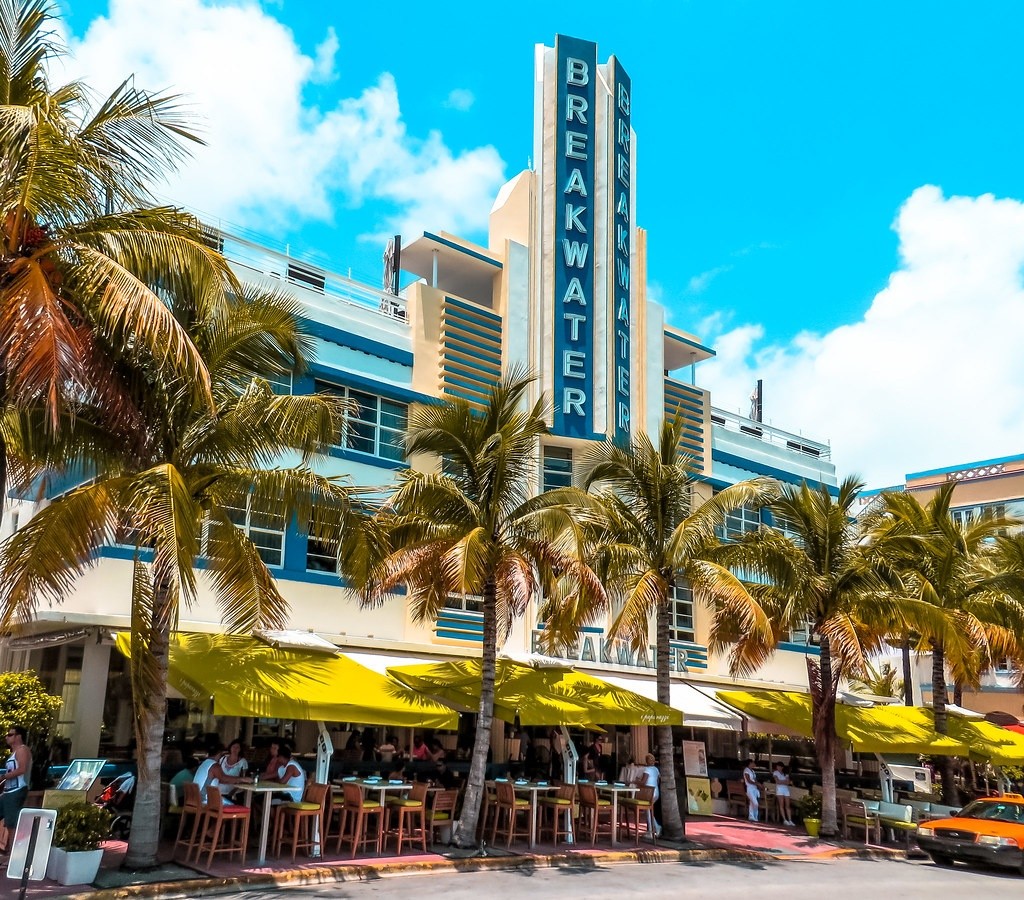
[593,674,1024,767]
[385,660,684,726]
[100,627,459,731]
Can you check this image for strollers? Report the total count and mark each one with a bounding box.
[92,771,136,842]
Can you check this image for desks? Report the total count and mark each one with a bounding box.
[332,777,413,852]
[486,780,561,850]
[584,783,641,848]
[234,781,301,866]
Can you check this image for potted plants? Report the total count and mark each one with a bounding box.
[798,790,822,836]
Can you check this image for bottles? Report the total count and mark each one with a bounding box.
[249,768,260,786]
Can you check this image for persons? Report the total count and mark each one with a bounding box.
[773,762,796,827]
[742,760,763,822]
[581,732,662,839]
[0,726,32,853]
[165,739,305,849]
[331,724,456,813]
[517,727,561,779]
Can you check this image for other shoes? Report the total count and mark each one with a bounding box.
[784,819,790,826]
[226,838,240,848]
[200,832,221,844]
[0,848,7,855]
[249,838,269,847]
[655,825,662,837]
[748,816,759,822]
[789,821,796,826]
[642,833,653,840]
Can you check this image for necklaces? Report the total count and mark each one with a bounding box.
[749,767,754,772]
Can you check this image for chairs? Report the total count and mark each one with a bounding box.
[839,797,880,846]
[725,779,748,822]
[758,789,780,823]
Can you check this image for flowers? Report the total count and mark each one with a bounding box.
[53,801,118,850]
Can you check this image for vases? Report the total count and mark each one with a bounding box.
[47,844,104,887]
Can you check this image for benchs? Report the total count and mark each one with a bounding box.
[881,818,917,848]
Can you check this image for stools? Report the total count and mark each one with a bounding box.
[159,782,657,871]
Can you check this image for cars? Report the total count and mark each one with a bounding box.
[917,795,1024,879]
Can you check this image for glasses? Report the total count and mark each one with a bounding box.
[3,732,14,737]
